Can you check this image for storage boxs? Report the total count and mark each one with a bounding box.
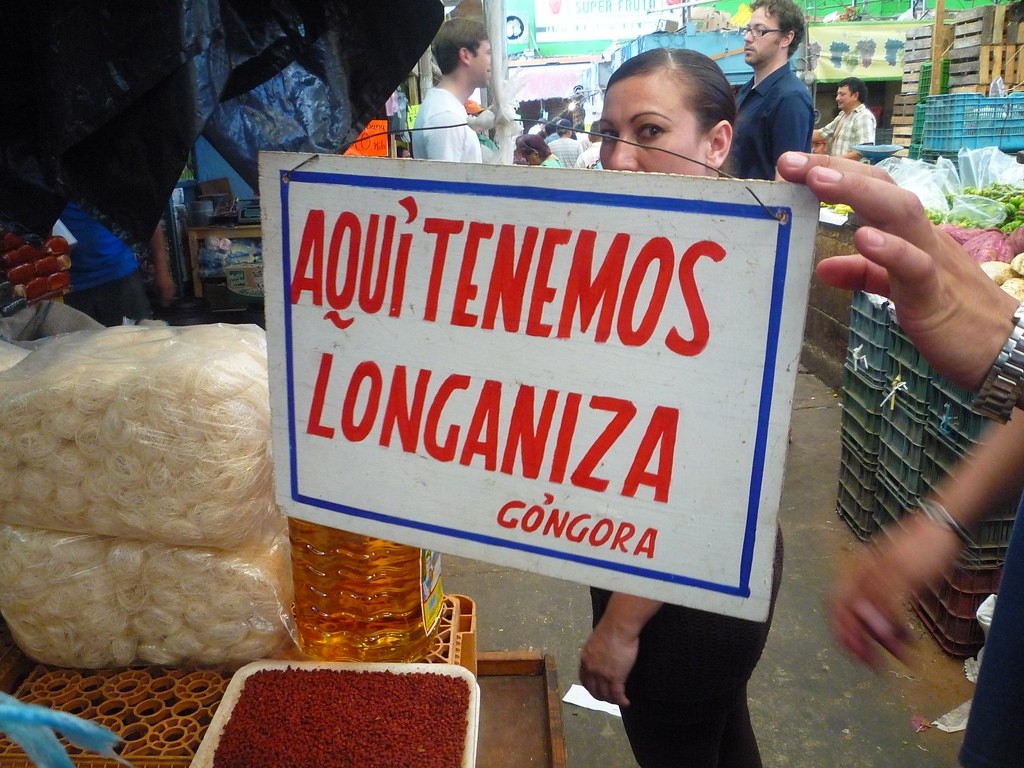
[833,90,1024,661]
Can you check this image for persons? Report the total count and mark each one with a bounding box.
[774,147,1024,768]
[806,75,878,167]
[42,196,180,330]
[577,45,789,768]
[461,100,603,174]
[411,18,494,163]
[395,146,411,159]
[809,139,828,154]
[727,0,817,186]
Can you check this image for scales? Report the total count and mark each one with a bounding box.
[853,143,905,165]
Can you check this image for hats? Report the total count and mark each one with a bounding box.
[556,118,572,128]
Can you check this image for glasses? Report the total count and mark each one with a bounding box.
[739,26,792,37]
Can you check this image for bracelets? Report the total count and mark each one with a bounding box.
[968,300,1024,426]
[922,500,976,550]
